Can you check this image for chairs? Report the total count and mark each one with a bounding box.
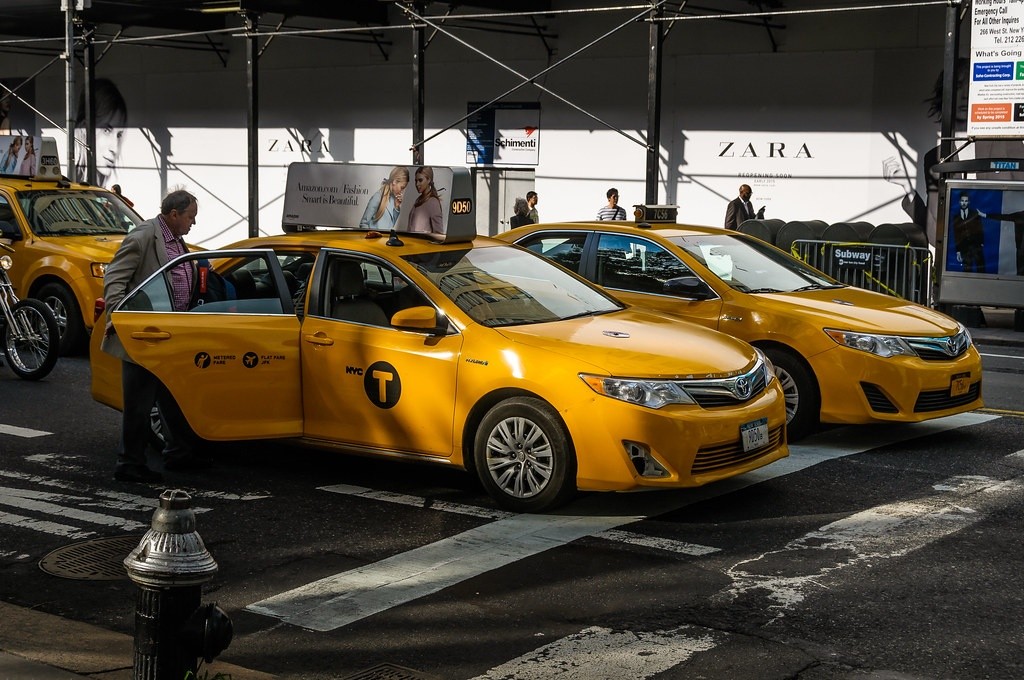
[232,263,315,315]
[330,260,390,327]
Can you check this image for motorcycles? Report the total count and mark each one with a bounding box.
[0,241,61,381]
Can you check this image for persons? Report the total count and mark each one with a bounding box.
[884,56,970,235]
[725,184,766,231]
[0,136,23,174]
[596,188,626,220]
[0,79,13,129]
[359,166,410,230]
[100,190,215,486]
[526,191,539,224]
[19,136,37,176]
[953,193,1024,276]
[72,77,128,186]
[102,185,134,220]
[510,197,535,230]
[407,166,443,235]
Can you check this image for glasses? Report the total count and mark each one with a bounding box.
[612,195,619,198]
[531,197,538,200]
[743,191,753,196]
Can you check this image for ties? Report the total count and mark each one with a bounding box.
[963,210,966,219]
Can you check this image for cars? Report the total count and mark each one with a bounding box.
[90,164,789,514]
[470,203,986,436]
[0,133,216,353]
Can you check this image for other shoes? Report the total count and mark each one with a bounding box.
[115,464,146,484]
